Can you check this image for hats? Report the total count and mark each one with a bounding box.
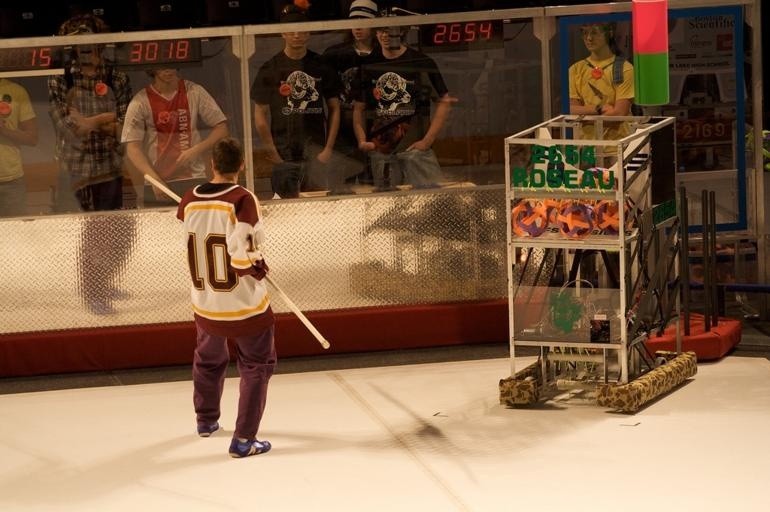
[349,1,378,19]
[279,4,309,23]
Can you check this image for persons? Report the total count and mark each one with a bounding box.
[0,74,37,216]
[324,1,380,161]
[353,6,450,186]
[46,26,135,210]
[123,66,230,205]
[566,19,636,165]
[250,5,340,164]
[177,139,271,458]
[80,214,137,316]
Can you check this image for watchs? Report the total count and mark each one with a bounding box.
[595,104,603,115]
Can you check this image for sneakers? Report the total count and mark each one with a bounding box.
[228,433,271,458]
[197,417,221,437]
